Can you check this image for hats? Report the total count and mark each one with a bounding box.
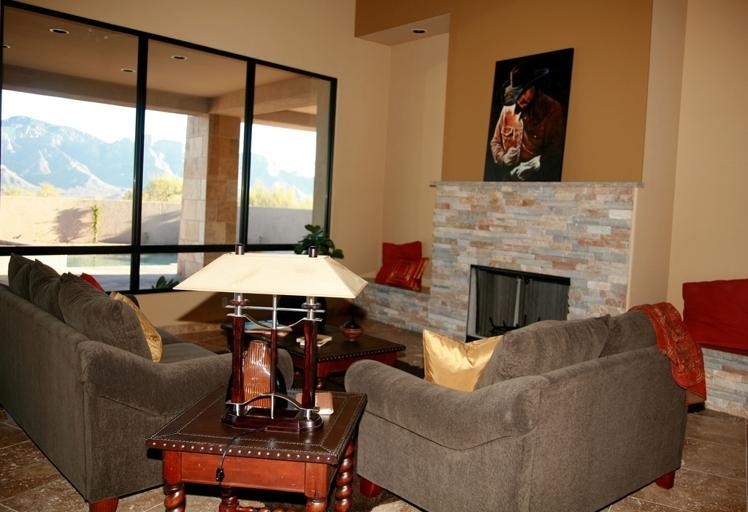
[503,60,550,106]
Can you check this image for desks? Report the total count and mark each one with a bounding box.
[146,384,367,512]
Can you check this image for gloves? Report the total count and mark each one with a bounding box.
[502,146,520,168]
[509,155,541,182]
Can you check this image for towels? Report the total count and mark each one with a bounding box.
[629,299,706,405]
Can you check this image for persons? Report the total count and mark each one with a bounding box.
[491,68,564,181]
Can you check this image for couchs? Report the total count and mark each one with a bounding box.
[0,282,294,512]
[342,347,685,512]
[120,291,283,350]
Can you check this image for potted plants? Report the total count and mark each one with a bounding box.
[278,225,344,332]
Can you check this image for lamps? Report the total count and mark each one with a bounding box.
[173,243,369,431]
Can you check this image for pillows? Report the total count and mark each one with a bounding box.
[382,252,430,292]
[374,241,422,289]
[422,302,677,392]
[6,252,163,363]
[679,278,748,355]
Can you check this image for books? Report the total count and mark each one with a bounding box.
[295,334,332,347]
[296,390,334,416]
